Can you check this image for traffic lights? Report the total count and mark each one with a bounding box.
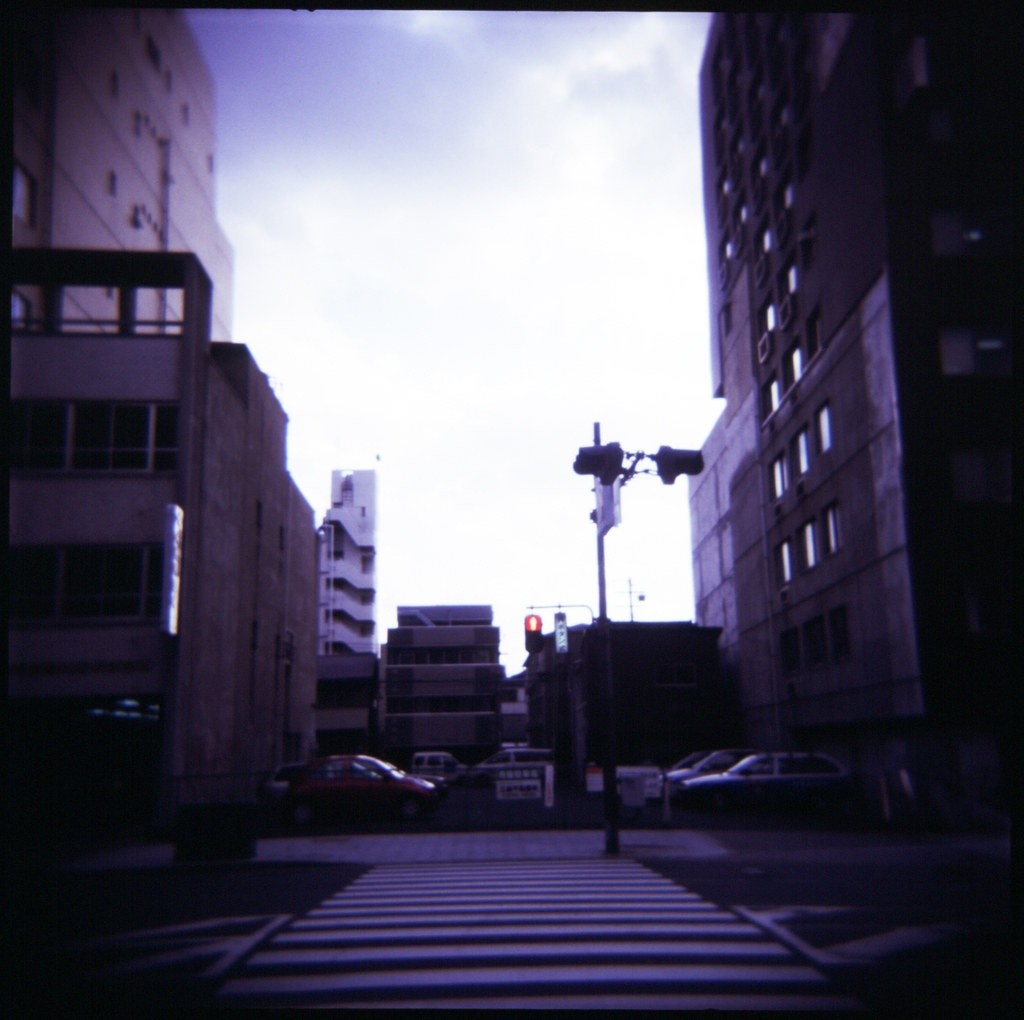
[572,442,624,484]
[657,444,707,484]
[525,614,543,653]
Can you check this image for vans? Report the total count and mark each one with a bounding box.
[410,751,469,787]
[466,748,555,785]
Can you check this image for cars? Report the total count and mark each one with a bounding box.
[647,749,766,805]
[268,755,451,830]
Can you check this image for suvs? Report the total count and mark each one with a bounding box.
[675,752,853,819]
